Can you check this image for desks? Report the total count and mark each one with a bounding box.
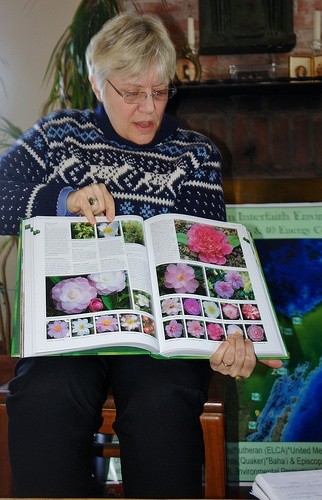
[169,76,322,112]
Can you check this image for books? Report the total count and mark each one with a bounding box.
[11,213,290,359]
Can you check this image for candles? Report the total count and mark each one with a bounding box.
[186,14,195,52]
[311,9,321,51]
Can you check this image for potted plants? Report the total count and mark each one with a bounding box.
[0,0,122,500]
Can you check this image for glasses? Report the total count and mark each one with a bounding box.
[106,78,177,104]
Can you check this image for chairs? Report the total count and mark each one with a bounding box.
[0,234,226,499]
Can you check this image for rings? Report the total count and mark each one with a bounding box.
[88,198,97,205]
[221,360,234,366]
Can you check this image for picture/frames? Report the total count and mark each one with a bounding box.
[289,55,322,83]
[173,50,200,83]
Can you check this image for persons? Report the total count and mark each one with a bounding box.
[0,11,283,499]
